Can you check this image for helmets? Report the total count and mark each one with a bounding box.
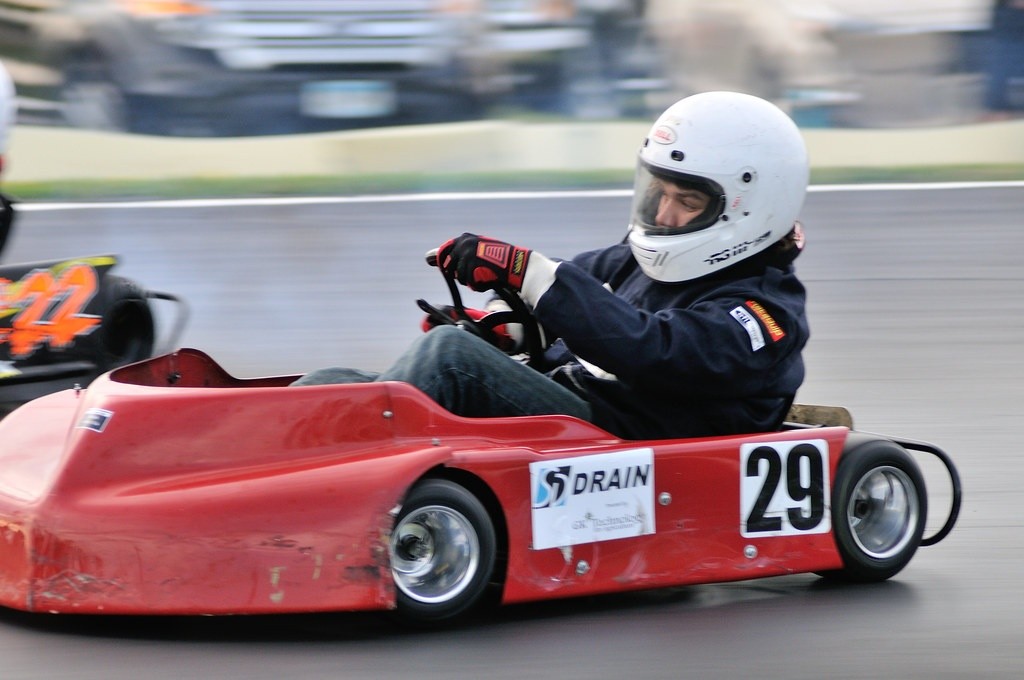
[0,62,17,172]
[628,91,808,282]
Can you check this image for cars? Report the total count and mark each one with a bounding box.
[446,1,594,120]
[1,1,480,135]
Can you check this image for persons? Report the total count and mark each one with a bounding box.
[288,90,811,440]
[0,63,16,175]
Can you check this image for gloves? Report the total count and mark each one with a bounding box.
[422,304,517,353]
[436,232,534,293]
